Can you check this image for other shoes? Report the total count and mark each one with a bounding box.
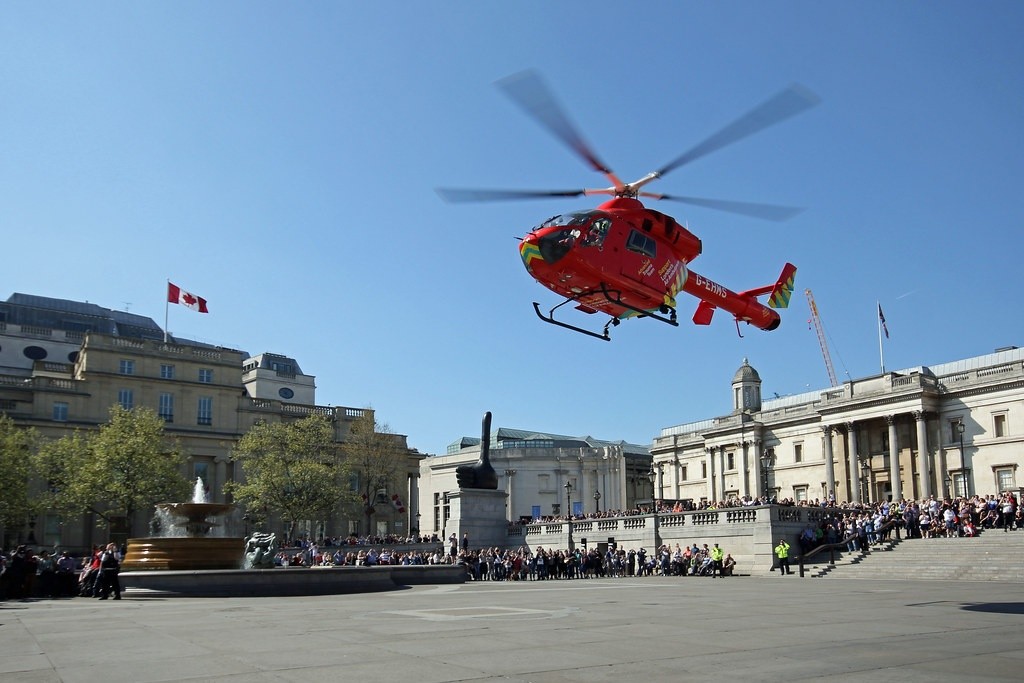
[98,596,108,600]
[113,596,121,600]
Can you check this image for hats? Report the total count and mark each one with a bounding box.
[714,544,719,546]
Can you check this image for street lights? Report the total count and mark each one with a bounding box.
[646,464,657,514]
[565,481,573,521]
[415,512,422,537]
[593,490,601,512]
[243,514,249,537]
[760,449,772,505]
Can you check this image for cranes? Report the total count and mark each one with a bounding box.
[805,288,851,386]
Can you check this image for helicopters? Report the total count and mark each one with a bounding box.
[430,71,827,343]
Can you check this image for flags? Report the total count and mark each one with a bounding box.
[879,303,888,338]
[168,282,208,313]
[393,494,405,513]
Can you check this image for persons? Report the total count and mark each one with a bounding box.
[449,533,457,565]
[505,491,860,522]
[459,532,736,582]
[274,532,451,565]
[774,538,791,575]
[800,492,1024,554]
[0,541,127,599]
